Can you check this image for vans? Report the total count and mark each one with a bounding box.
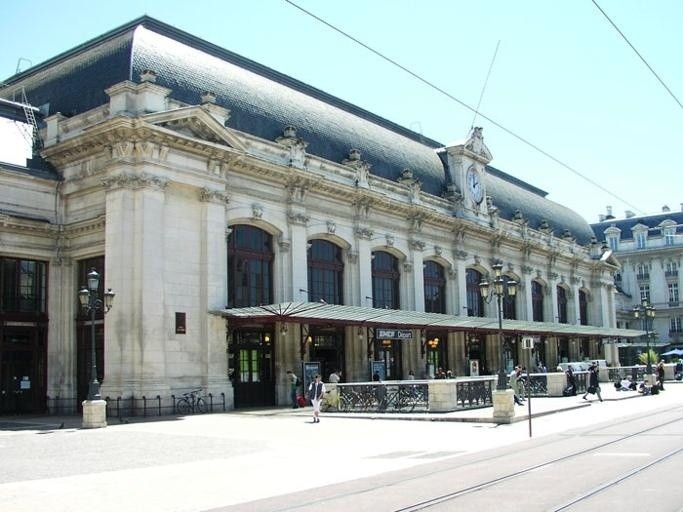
[586,359,610,368]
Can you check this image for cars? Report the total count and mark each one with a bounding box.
[560,362,600,372]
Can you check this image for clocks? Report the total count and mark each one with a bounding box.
[466,164,482,203]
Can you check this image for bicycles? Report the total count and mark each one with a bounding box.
[609,370,620,381]
[178,389,208,414]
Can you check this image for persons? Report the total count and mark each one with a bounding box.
[566,362,602,401]
[329,369,340,383]
[542,364,547,372]
[308,375,326,422]
[517,366,528,401]
[286,370,299,408]
[509,367,523,406]
[408,370,414,380]
[373,371,380,381]
[614,359,682,395]
[434,367,446,379]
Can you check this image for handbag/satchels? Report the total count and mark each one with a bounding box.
[588,387,596,394]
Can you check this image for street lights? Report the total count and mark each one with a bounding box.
[478,258,519,421]
[429,338,440,376]
[632,295,657,385]
[78,265,114,429]
